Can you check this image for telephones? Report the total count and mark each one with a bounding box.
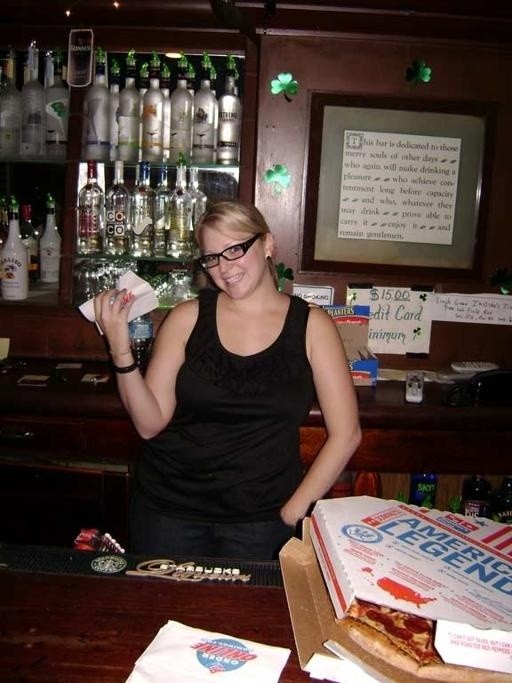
[467,370,512,393]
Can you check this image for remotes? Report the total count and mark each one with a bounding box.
[450,359,498,375]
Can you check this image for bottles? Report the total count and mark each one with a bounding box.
[0,38,70,301]
[77,47,243,373]
[393,473,512,525]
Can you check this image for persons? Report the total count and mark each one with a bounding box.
[92,200,364,560]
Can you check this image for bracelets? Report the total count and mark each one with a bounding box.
[108,347,131,356]
[113,360,139,374]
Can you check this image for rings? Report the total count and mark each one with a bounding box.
[108,296,117,305]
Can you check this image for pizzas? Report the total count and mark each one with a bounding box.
[335,598,512,683]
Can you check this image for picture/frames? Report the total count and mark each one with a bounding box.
[301,92,499,282]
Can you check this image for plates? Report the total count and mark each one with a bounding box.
[452,360,500,374]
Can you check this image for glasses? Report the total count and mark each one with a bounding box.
[197,232,262,271]
